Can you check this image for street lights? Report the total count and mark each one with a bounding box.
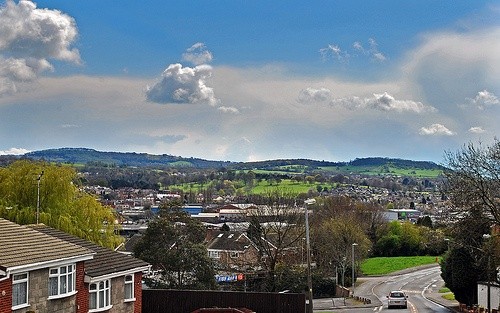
[305,198,319,313]
[350,243,359,298]
[443,236,479,269]
[242,245,250,294]
[464,243,493,282]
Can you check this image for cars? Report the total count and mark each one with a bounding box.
[385,291,409,308]
